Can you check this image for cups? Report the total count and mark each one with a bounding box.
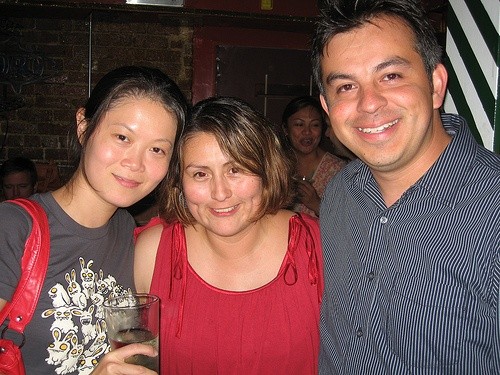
[102,293,159,375]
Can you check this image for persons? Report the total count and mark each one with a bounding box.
[0,158,40,200]
[133,97,324,375]
[0,65,189,375]
[305,0,500,375]
[279,95,358,218]
